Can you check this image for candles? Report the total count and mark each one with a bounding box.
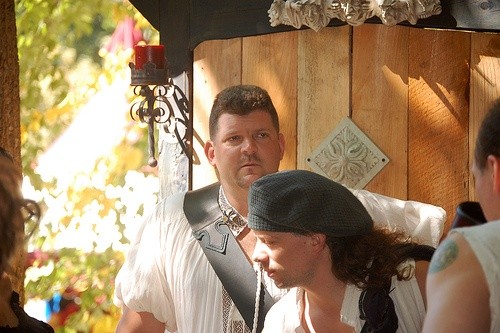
[134,44,164,68]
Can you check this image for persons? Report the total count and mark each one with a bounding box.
[111,86,285,333]
[423,98,500,333]
[0,148,54,333]
[246,170,435,333]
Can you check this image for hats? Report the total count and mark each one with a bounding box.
[247,170,374,241]
